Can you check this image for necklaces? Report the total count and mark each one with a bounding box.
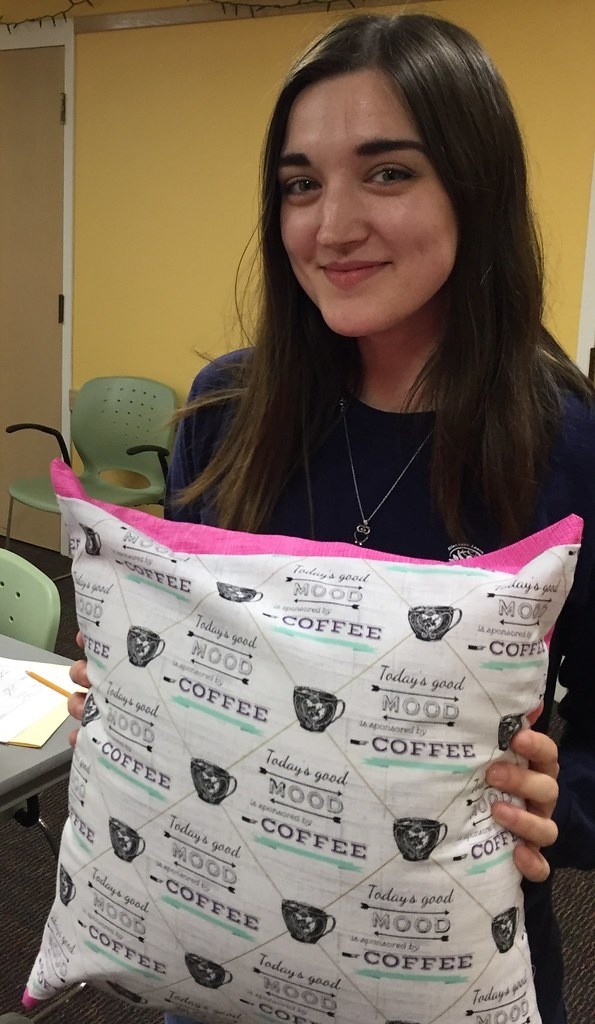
[335,394,435,546]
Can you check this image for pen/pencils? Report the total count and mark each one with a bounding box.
[25,669,72,699]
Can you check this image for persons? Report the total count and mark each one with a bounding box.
[70,12,594,1024]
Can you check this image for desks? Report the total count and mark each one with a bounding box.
[0,633,93,824]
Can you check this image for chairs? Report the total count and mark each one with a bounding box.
[0,547,60,863]
[0,377,180,584]
